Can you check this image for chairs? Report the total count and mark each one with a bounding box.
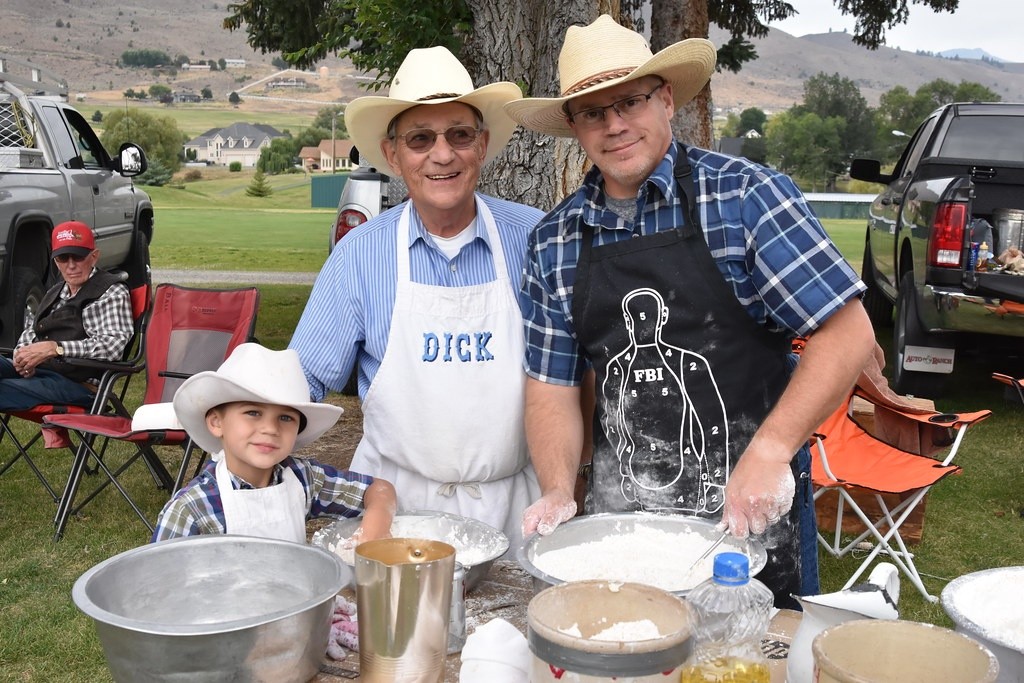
[792,331,991,604]
[0,283,153,533]
[39,282,261,546]
[992,372,1024,403]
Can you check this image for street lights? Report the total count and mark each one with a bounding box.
[332,111,343,175]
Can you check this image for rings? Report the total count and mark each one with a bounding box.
[26,370,29,373]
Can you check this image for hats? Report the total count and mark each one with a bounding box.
[344,46,525,179]
[173,342,345,453]
[503,14,717,137]
[51,220,96,259]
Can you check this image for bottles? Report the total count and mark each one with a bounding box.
[976,240,989,272]
[679,552,774,683]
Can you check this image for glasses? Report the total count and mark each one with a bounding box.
[568,85,662,125]
[388,125,482,152]
[55,253,85,263]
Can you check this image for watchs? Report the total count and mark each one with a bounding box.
[55,340,65,360]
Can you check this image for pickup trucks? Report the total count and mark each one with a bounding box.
[329,145,390,258]
[1,93,153,345]
[851,102,1023,399]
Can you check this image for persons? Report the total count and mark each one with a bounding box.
[152,342,399,552]
[284,46,553,564]
[0,221,136,416]
[504,14,878,612]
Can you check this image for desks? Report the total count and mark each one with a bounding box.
[301,560,803,683]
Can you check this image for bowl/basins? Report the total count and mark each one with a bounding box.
[939,564,1023,682]
[812,618,999,683]
[517,510,768,600]
[313,511,509,593]
[72,533,352,683]
[528,580,693,683]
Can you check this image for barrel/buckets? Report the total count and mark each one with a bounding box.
[354,539,455,683]
[991,206,1024,259]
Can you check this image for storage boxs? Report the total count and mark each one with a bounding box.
[0,146,44,169]
[815,396,934,542]
